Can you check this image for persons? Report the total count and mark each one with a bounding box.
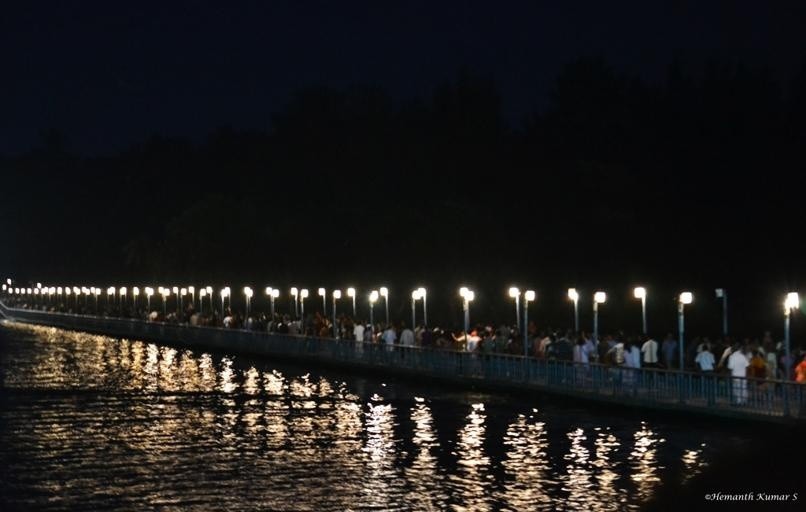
[5,296,806,405]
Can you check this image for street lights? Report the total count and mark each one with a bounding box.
[782,290,800,380]
[632,285,647,335]
[675,289,695,380]
[715,286,729,338]
